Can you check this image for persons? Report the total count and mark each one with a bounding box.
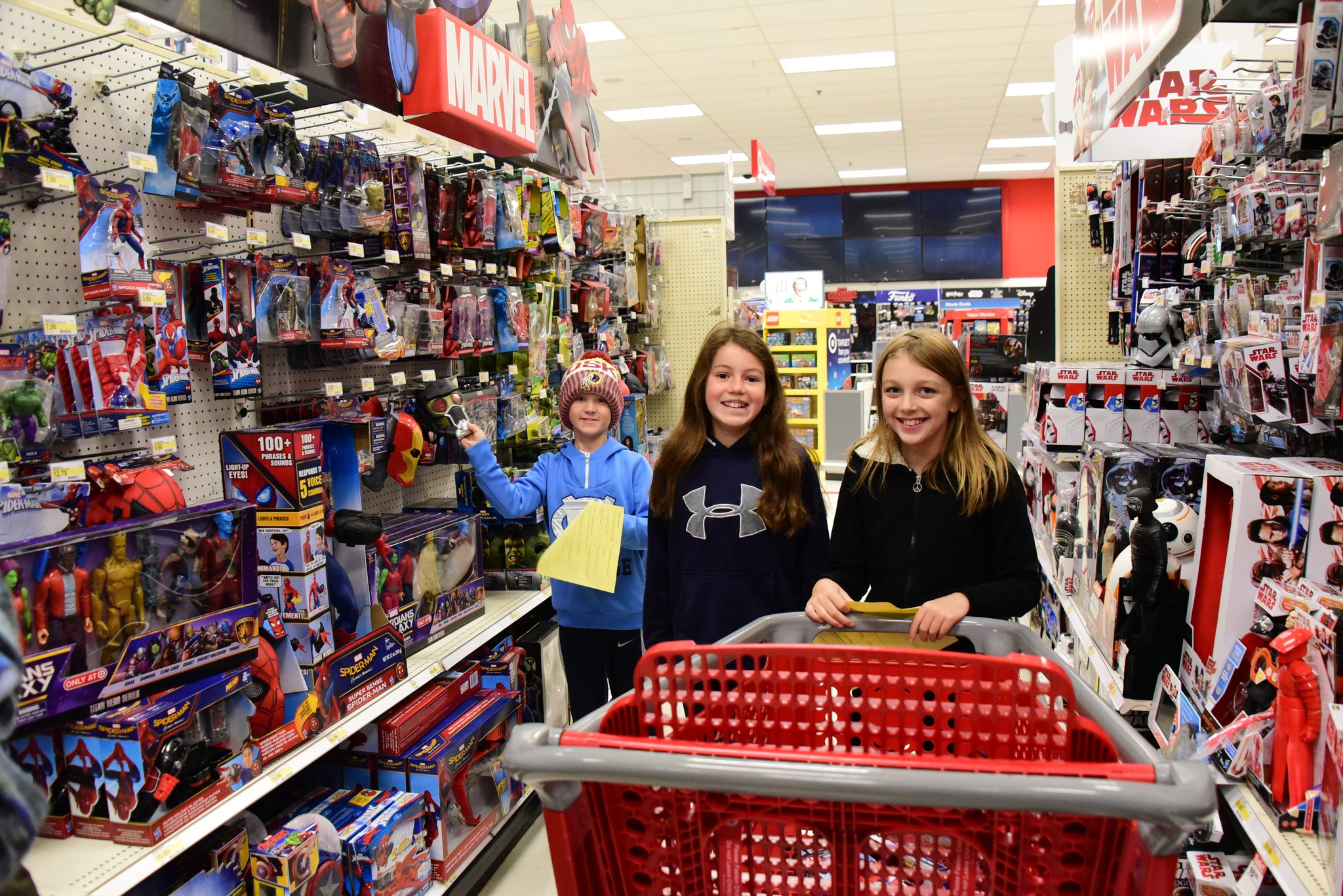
[1056,490,1321,815]
[265,525,325,572]
[784,278,815,302]
[1244,481,1342,586]
[2,510,238,662]
[1251,96,1319,416]
[323,472,421,652]
[805,330,1041,755]
[462,350,651,737]
[641,326,831,742]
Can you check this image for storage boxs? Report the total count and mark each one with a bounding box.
[968,382,1026,454]
[1006,0,1343,896]
[766,331,817,448]
[0,0,678,896]
[720,817,982,896]
[953,331,1025,383]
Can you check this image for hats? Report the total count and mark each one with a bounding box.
[558,351,624,431]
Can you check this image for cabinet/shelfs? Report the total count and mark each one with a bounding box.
[390,582,561,896]
[17,660,487,896]
[1056,162,1160,719]
[763,310,828,464]
